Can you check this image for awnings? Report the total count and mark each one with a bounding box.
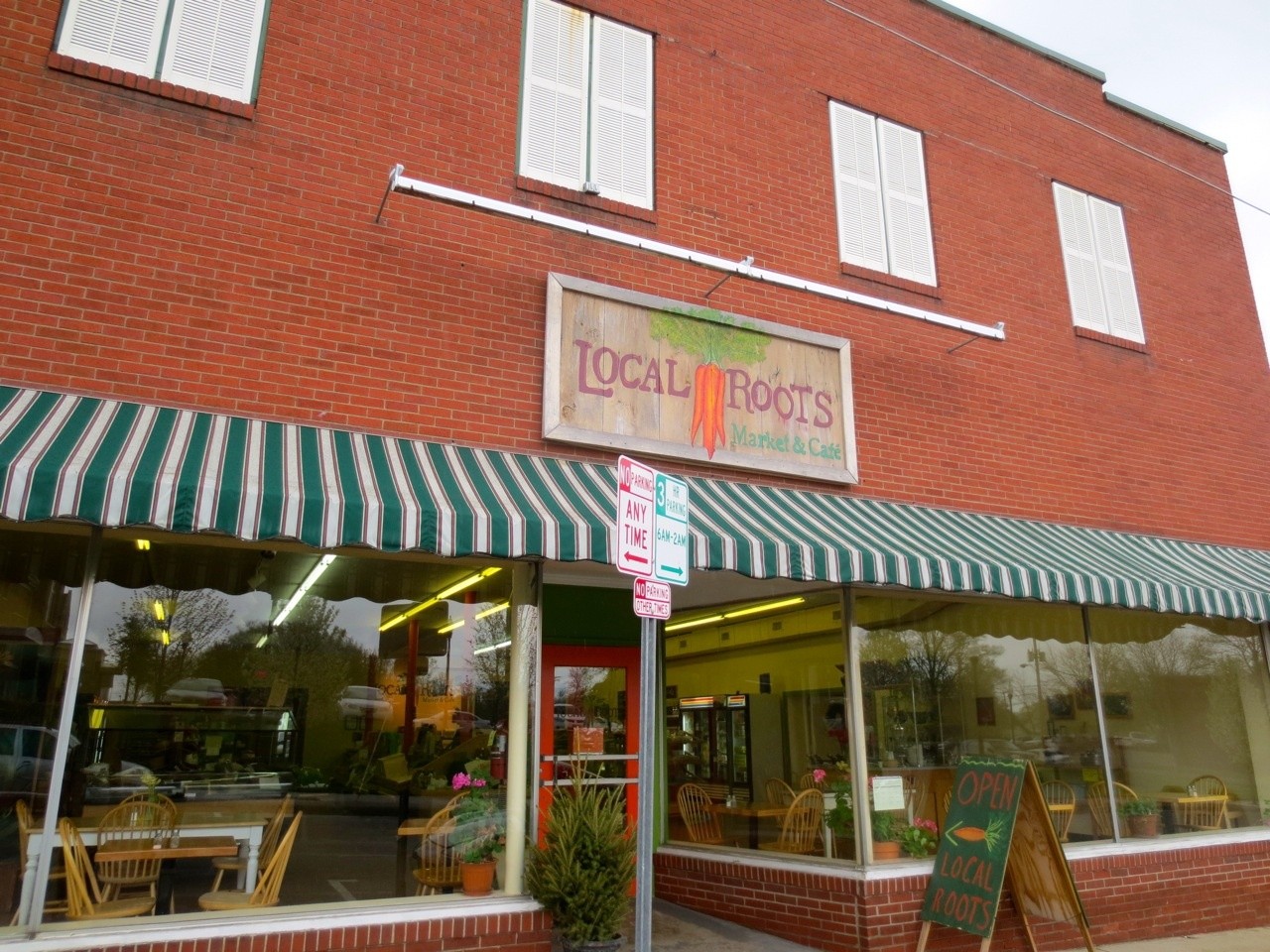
[0,385,1269,626]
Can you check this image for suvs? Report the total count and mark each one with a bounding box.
[0,724,156,793]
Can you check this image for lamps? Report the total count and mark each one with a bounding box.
[132,539,512,657]
[663,597,807,632]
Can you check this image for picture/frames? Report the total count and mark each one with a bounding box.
[1043,675,1134,722]
[976,696,997,728]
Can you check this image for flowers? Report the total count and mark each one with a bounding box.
[444,758,506,864]
[812,766,827,784]
[897,816,938,858]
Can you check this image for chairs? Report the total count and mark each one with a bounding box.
[800,775,829,789]
[764,779,825,849]
[676,784,738,849]
[895,776,924,838]
[409,803,479,899]
[756,787,822,855]
[1038,771,1243,844]
[13,791,305,922]
[442,792,487,813]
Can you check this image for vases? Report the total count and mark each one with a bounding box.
[457,858,498,898]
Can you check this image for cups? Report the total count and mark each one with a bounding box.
[1187,784,1197,797]
[726,792,739,809]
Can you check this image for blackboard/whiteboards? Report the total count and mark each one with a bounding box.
[921,754,1090,939]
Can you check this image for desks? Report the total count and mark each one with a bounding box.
[781,789,920,857]
[395,818,481,892]
[700,800,809,852]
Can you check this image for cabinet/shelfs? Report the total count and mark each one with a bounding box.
[881,682,967,763]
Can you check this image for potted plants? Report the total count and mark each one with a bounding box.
[522,729,640,951]
[869,810,900,861]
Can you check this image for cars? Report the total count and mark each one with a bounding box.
[165,677,227,708]
[553,704,584,729]
[415,709,490,731]
[954,731,1151,766]
[336,685,393,719]
[594,717,623,733]
